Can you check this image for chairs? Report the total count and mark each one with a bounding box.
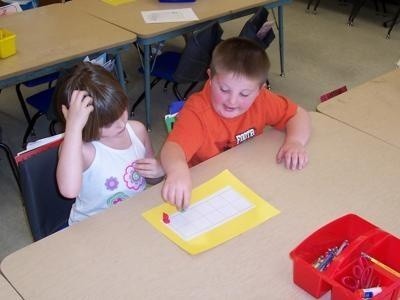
[13,140,92,242]
[13,3,122,147]
[130,7,277,118]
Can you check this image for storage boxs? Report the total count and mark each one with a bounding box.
[281,211,399,299]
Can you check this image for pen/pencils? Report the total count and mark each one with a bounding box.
[312,240,350,271]
[361,252,400,278]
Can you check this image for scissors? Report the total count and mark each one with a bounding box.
[342,256,381,290]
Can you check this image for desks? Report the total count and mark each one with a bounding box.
[317,62,400,161]
[0,110,398,300]
[69,1,288,135]
[0,3,139,137]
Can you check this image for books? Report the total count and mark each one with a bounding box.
[256,19,275,40]
[164,112,179,136]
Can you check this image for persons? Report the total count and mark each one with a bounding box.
[54,61,165,226]
[160,37,311,211]
